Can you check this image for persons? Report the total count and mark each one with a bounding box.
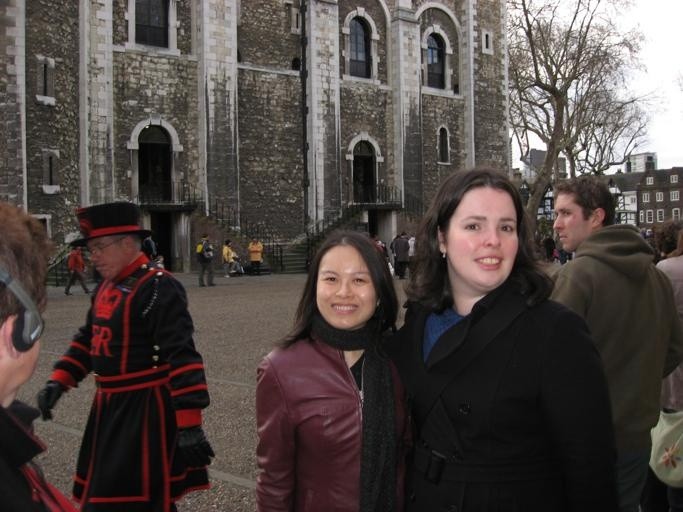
[194,233,217,286]
[63,245,95,296]
[398,164,623,510]
[256,228,400,509]
[635,220,682,257]
[36,200,214,511]
[541,172,683,512]
[641,224,683,512]
[0,201,79,511]
[532,229,575,267]
[221,238,243,274]
[368,230,418,281]
[247,236,264,276]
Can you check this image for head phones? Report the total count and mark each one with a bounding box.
[0,268,45,352]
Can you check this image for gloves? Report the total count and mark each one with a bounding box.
[35,381,65,422]
[175,426,215,468]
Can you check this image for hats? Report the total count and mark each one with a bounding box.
[68,201,152,247]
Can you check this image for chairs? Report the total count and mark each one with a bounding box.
[245,256,271,276]
